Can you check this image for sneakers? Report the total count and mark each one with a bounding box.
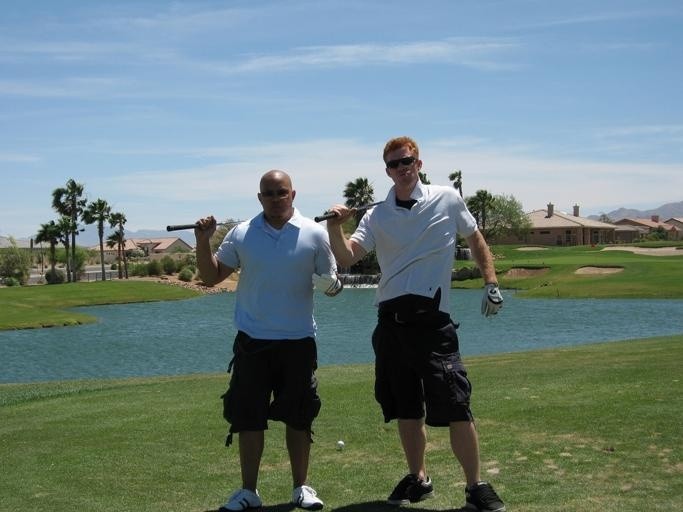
[464,480,506,512]
[386,473,434,505]
[223,487,261,511]
[292,484,324,510]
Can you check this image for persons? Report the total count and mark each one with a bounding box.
[327,136,506,512]
[194,169,342,512]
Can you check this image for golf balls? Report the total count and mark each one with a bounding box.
[337,441,344,448]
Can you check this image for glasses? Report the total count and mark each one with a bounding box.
[386,156,417,169]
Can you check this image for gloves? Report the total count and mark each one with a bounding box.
[310,272,343,296]
[480,282,504,318]
